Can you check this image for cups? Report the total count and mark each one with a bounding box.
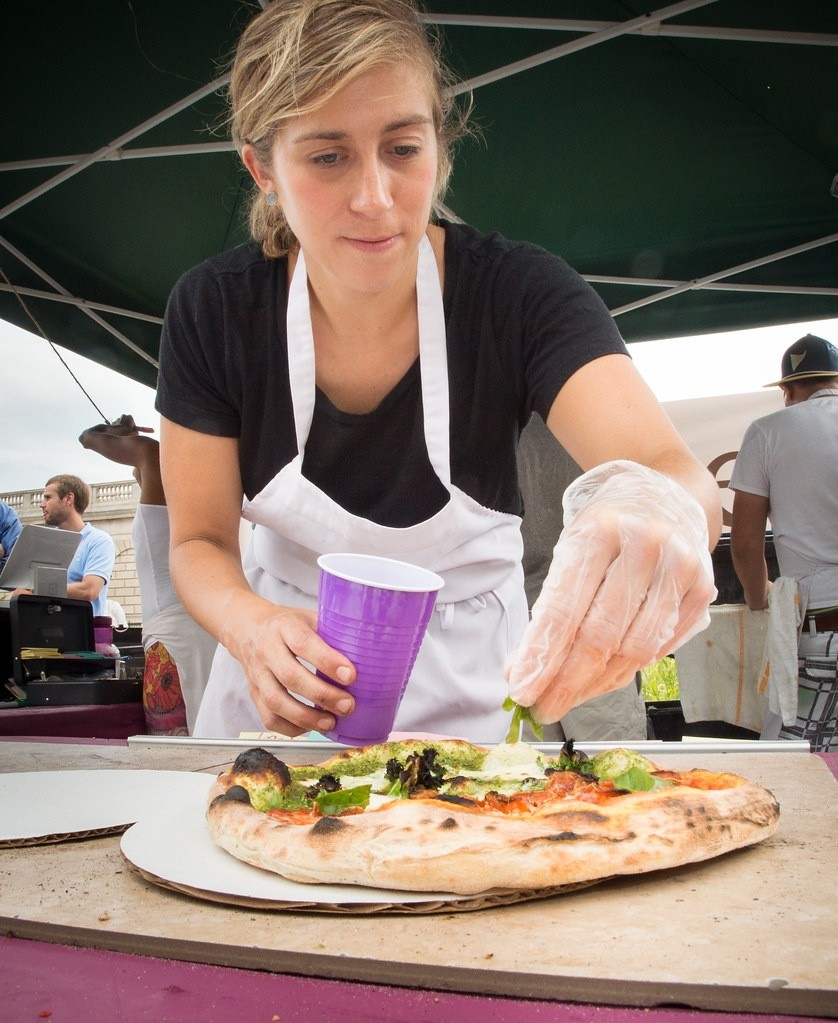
[309,552,447,745]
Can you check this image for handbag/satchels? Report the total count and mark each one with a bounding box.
[103,598,129,632]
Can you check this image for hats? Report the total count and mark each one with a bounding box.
[761,333,838,388]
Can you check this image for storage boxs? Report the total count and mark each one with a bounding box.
[648,703,750,740]
[9,593,141,707]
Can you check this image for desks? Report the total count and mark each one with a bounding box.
[0,736,838,1023]
[0,704,145,739]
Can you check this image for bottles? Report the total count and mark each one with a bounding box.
[94,616,113,656]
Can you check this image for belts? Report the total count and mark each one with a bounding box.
[797,604,838,632]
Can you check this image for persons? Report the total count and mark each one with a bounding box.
[11,473,115,616]
[515,410,647,741]
[155,0,726,747]
[79,414,218,736]
[729,333,838,752]
[0,499,24,575]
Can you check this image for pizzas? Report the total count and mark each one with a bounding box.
[208,739,781,894]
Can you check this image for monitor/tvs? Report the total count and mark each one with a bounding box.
[0,525,82,597]
